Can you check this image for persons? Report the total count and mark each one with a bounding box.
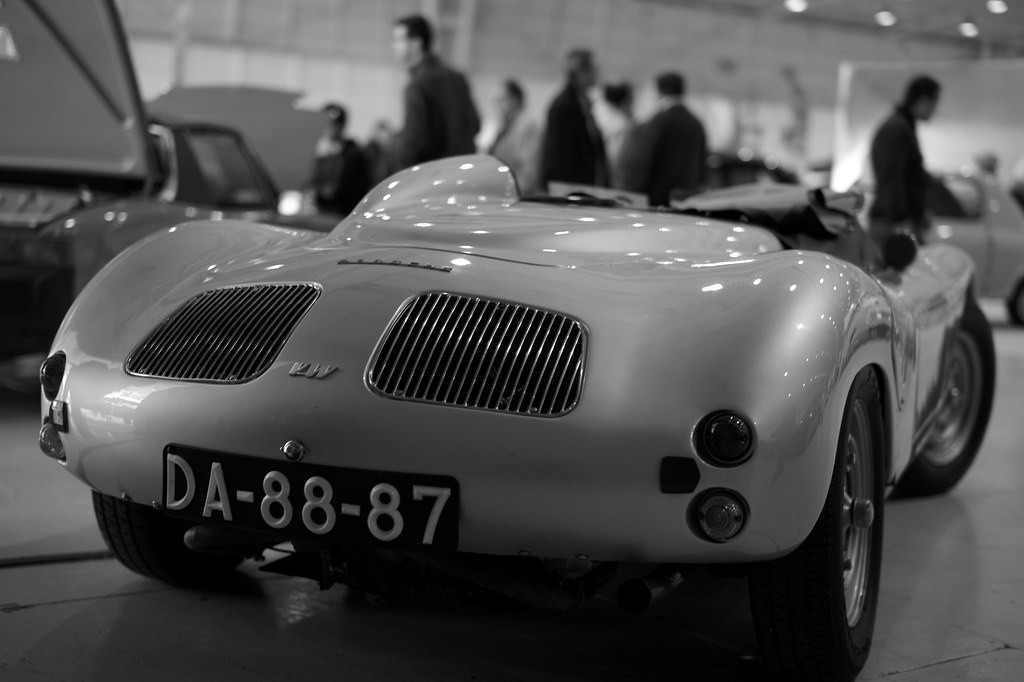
[615,71,707,206]
[867,73,979,253]
[374,13,482,172]
[482,76,543,199]
[539,49,612,196]
[591,80,637,170]
[311,105,377,217]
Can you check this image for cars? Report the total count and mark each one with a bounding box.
[0,0,341,390]
[666,158,1024,329]
[39,152,996,682]
